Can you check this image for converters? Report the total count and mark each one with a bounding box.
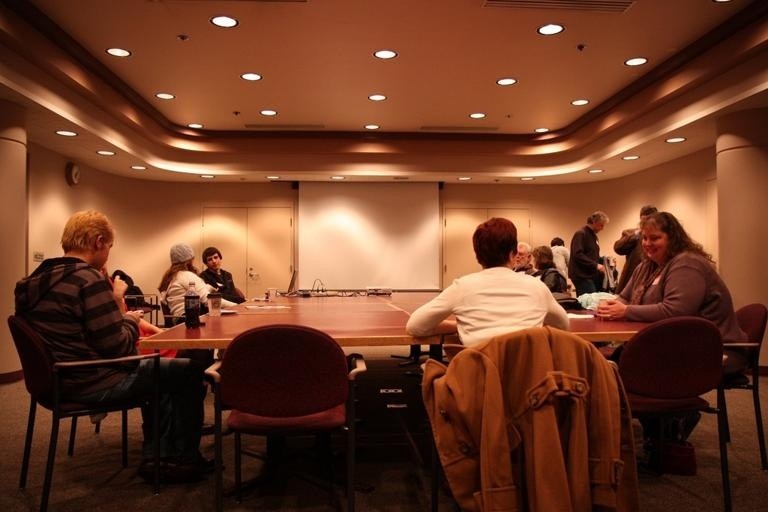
[302,289,310,297]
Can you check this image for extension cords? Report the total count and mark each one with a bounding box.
[302,290,336,297]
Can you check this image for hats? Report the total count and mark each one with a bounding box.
[170,243,194,263]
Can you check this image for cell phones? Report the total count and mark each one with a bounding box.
[132,310,144,314]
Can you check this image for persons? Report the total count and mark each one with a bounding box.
[406,218,569,349]
[594,212,744,475]
[197,247,242,304]
[515,205,664,301]
[158,242,249,323]
[103,270,181,360]
[12,210,211,486]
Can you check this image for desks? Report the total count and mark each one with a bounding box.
[137,289,651,510]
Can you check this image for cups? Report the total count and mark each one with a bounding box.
[207,293,223,318]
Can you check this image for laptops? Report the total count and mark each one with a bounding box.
[278,270,297,294]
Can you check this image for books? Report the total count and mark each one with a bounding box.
[221,309,239,314]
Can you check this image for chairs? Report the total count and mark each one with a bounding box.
[391,328,618,510]
[614,316,733,511]
[726,301,768,474]
[204,323,367,509]
[7,261,767,328]
[6,316,161,512]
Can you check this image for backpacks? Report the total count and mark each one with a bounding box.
[112,270,144,307]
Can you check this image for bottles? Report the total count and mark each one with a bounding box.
[183,281,202,329]
